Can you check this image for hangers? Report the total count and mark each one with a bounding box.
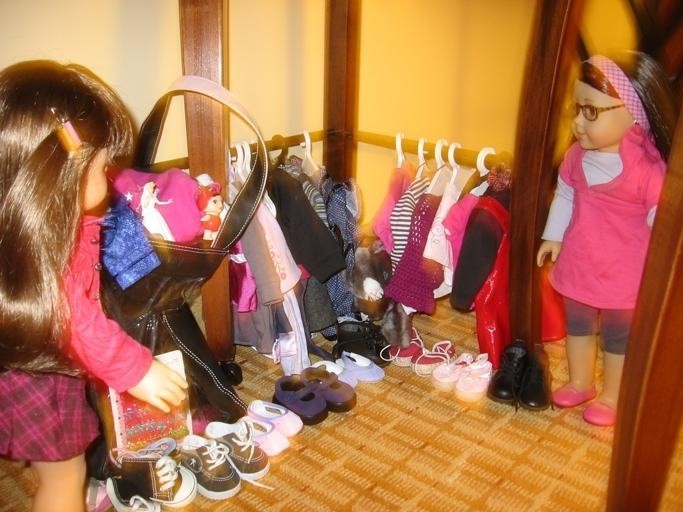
[386,126,503,193]
[222,129,323,193]
